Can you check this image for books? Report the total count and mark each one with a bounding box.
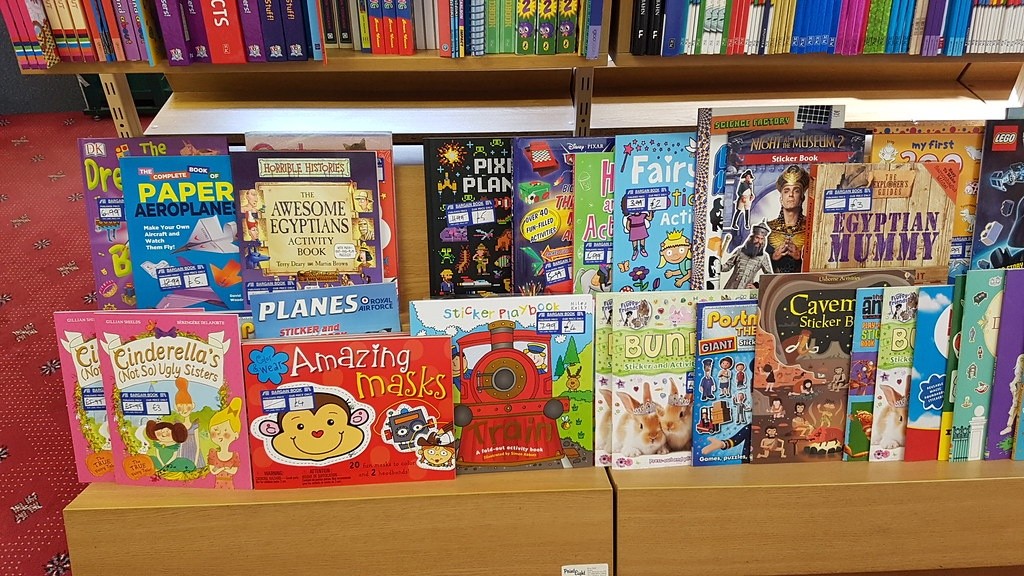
[53,106,1024,489]
[0,1,1024,68]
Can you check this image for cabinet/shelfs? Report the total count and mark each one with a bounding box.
[0,1,1024,576]
